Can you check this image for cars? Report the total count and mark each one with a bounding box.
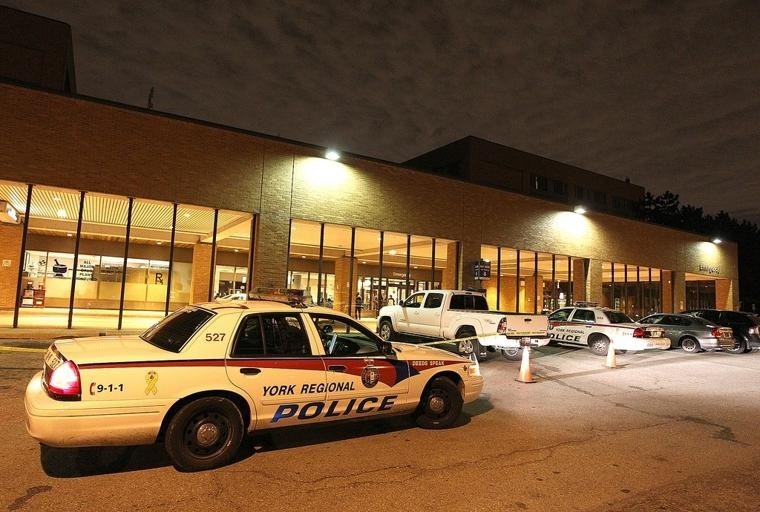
[219,292,247,301]
[631,311,739,354]
[11,286,488,480]
[537,299,672,358]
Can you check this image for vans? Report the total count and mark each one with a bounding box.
[677,307,760,356]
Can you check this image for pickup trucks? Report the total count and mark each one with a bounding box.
[370,286,556,362]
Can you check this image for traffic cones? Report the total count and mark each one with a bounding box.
[604,340,623,369]
[513,344,536,386]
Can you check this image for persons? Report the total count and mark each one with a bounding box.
[388,295,395,306]
[355,292,362,320]
[325,298,333,308]
